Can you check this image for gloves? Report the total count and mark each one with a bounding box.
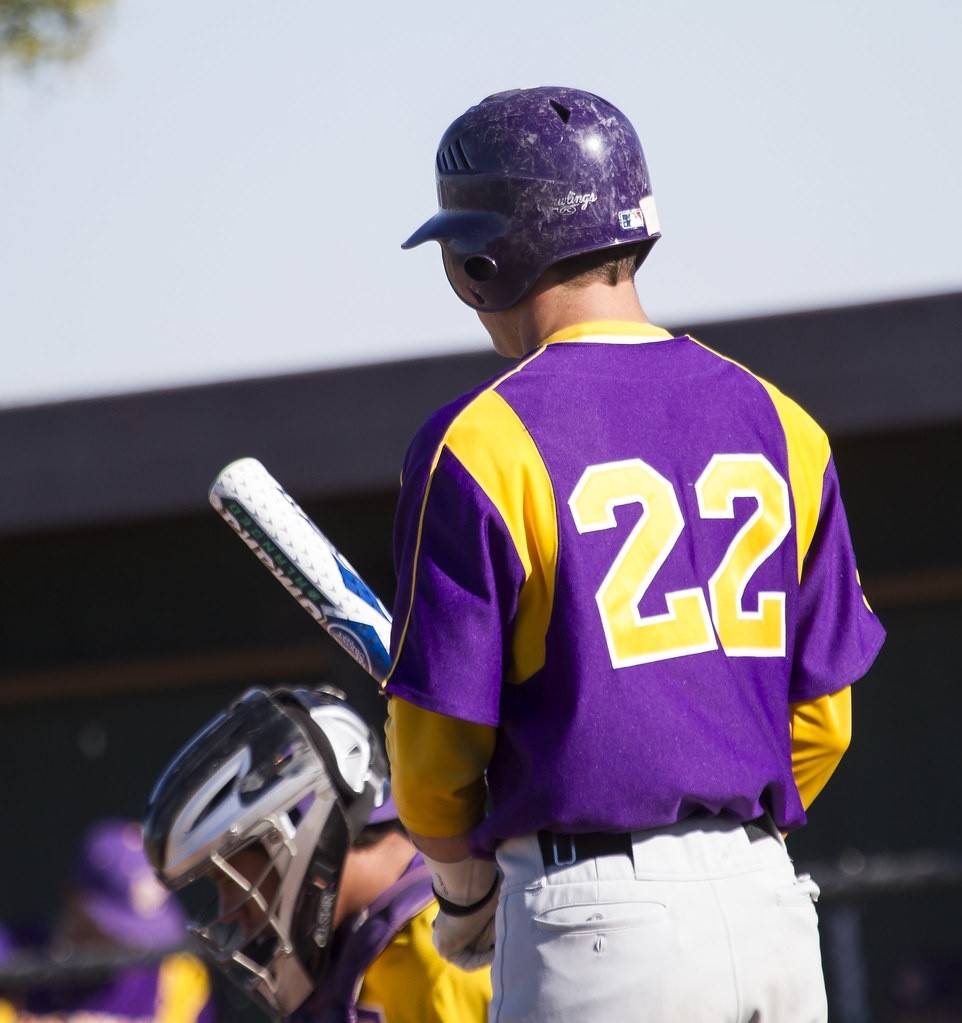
[411,839,504,974]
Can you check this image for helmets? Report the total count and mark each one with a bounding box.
[141,688,399,1023]
[401,86,661,313]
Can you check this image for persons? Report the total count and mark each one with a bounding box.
[380,86,887,1023]
[0,824,214,1023]
[142,687,493,1023]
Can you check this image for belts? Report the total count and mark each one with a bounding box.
[536,812,779,866]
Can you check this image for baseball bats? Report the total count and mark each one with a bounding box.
[207,456,393,686]
